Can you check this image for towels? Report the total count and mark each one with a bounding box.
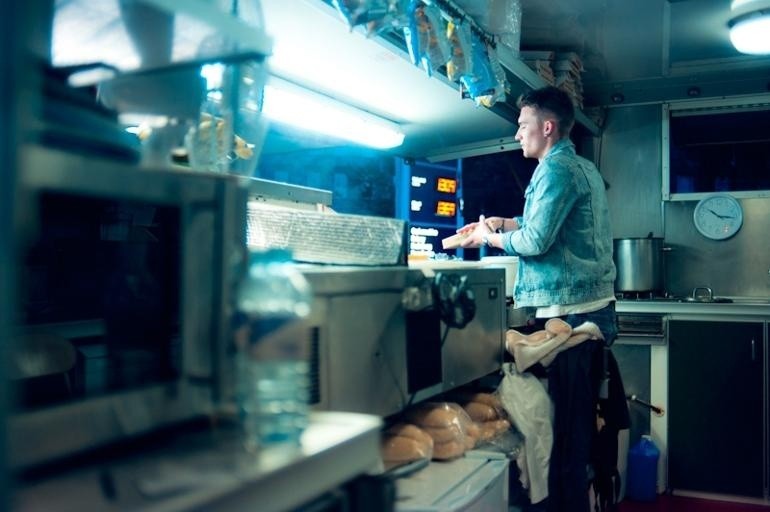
[503,316,572,374]
[537,321,605,368]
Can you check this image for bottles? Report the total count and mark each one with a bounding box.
[246,246,312,443]
[235,251,264,435]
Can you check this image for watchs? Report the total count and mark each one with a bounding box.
[482,234,492,250]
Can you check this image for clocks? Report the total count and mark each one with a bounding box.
[693,194,744,240]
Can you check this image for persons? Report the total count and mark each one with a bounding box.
[456,85,620,512]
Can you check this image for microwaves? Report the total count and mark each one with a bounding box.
[0,147,250,476]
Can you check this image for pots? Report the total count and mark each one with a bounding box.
[612,237,672,293]
